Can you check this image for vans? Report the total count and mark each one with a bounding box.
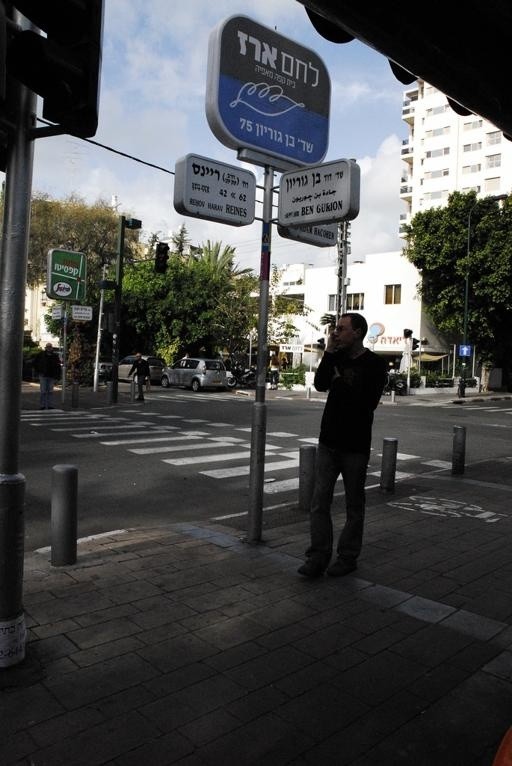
[159,356,229,391]
[117,353,168,387]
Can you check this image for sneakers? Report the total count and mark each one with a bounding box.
[328,553,358,576]
[297,558,326,578]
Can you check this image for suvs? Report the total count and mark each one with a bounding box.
[51,347,71,364]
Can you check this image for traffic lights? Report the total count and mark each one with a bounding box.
[153,241,170,274]
[413,337,420,349]
[3,0,107,138]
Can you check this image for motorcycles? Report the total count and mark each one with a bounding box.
[225,360,258,389]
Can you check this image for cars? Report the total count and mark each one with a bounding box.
[92,355,114,381]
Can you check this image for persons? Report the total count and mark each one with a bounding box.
[297,311,389,579]
[267,353,280,391]
[30,342,61,411]
[180,352,190,368]
[126,351,151,401]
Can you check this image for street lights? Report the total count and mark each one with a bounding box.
[99,212,143,406]
[456,192,507,397]
